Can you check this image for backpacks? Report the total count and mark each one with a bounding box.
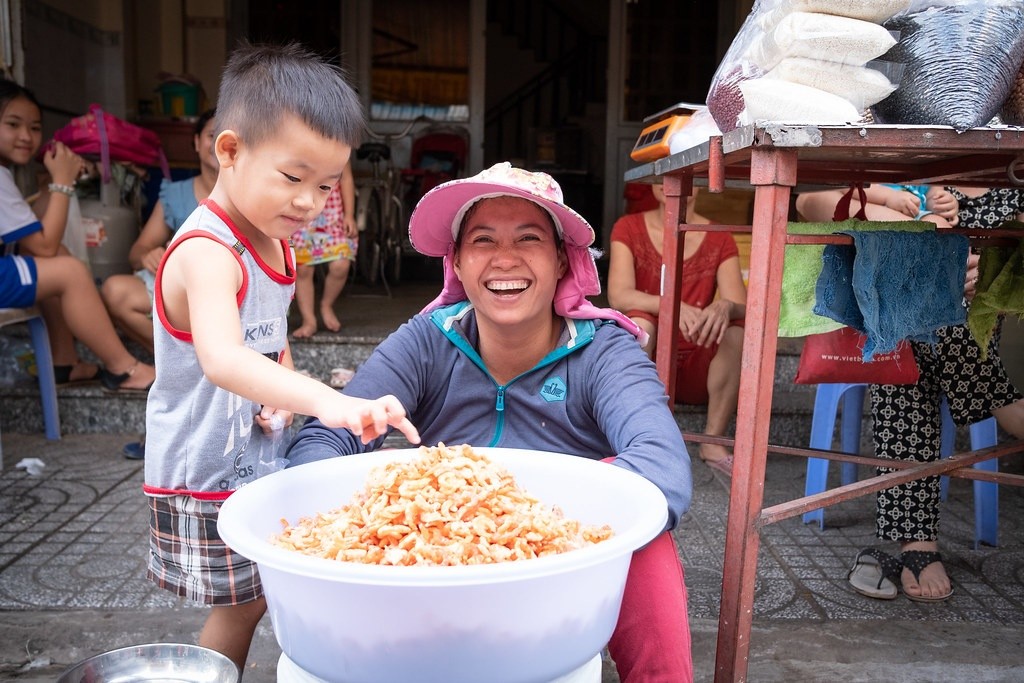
[39,102,171,184]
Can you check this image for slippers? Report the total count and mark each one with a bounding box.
[847,548,898,600]
[704,452,733,477]
[122,434,145,460]
[898,551,956,603]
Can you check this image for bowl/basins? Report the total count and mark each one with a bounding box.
[217,450,668,683]
[56,641,241,683]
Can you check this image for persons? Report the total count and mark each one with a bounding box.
[99,106,216,351]
[0,79,157,395]
[138,38,422,680]
[605,161,750,482]
[790,177,1024,600]
[276,158,696,683]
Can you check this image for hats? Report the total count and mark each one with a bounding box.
[408,162,649,349]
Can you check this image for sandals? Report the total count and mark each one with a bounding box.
[52,359,104,382]
[99,358,157,391]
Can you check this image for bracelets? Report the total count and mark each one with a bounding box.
[48,183,74,197]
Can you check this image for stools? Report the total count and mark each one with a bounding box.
[803,383,1000,550]
[0,308,61,443]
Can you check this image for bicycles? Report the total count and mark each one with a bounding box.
[344,106,441,302]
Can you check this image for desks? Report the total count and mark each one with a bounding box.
[625,123,1024,683]
[133,120,201,168]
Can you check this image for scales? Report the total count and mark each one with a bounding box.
[630,103,702,163]
